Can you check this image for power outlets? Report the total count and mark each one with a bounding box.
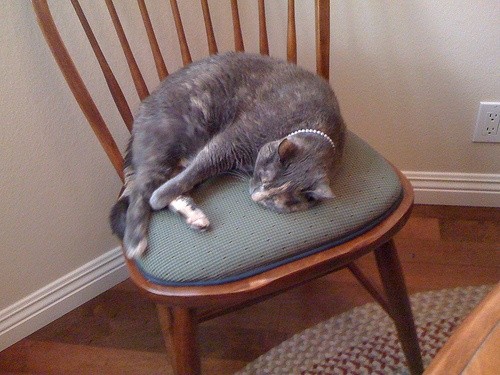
[472,100,500,144]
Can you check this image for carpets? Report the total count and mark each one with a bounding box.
[231,283,495,375]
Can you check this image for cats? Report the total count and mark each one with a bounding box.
[107,49,348,259]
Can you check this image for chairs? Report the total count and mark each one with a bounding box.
[31,0,425,375]
